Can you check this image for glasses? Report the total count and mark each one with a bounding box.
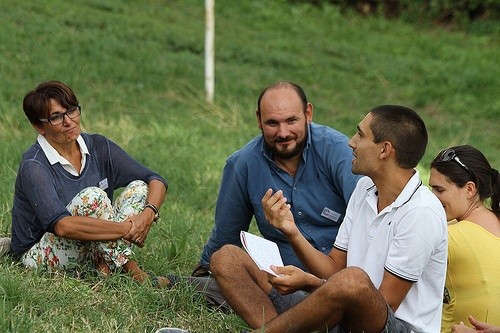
[39,104,82,127]
[437,146,476,179]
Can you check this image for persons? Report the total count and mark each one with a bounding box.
[13,81,168,291]
[452,315,500,333]
[152,83,368,314]
[211,105,448,333]
[428,145,500,333]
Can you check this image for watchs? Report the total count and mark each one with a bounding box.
[145,203,159,222]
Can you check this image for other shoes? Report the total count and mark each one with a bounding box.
[150,275,184,292]
